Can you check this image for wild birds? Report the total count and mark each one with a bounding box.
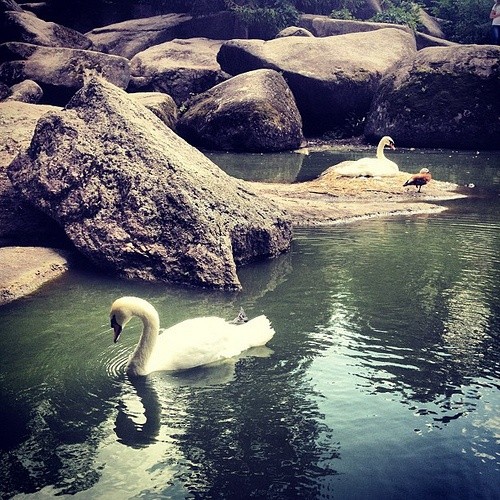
[403,167,432,194]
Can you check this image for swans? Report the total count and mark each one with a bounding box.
[109,293,276,377]
[321,136,400,177]
